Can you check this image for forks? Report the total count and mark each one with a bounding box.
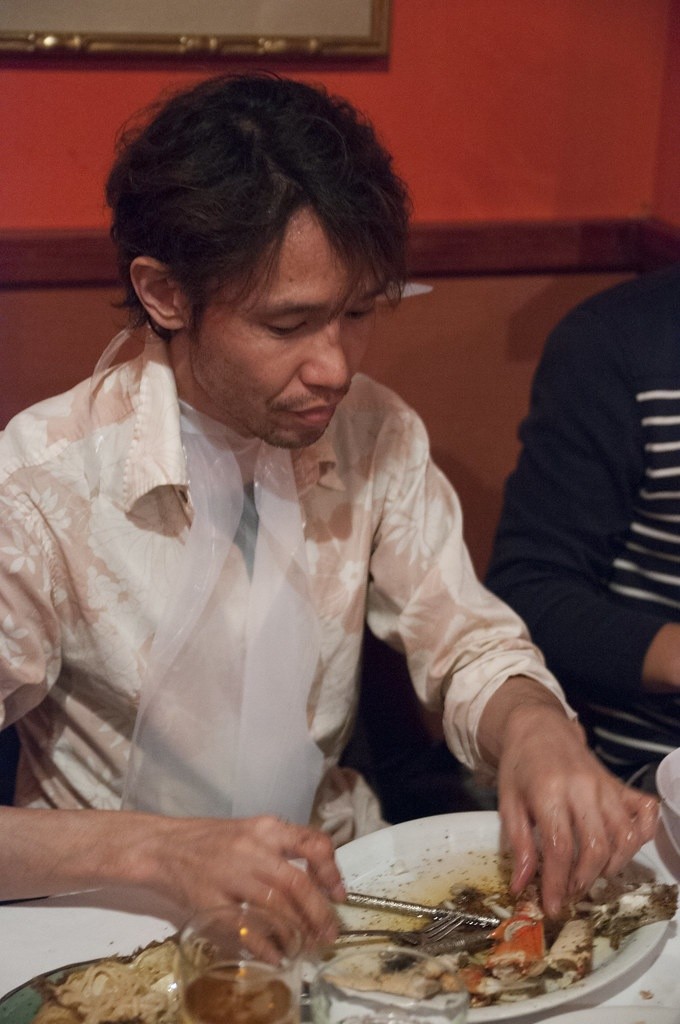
[333,913,463,946]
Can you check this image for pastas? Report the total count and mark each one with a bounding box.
[53,938,209,1024]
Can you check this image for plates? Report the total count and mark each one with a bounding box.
[659,797,679,860]
[0,954,305,1024]
[269,809,671,1022]
[655,746,680,818]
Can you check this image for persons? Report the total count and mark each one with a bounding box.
[485,269,680,799]
[0,74,658,970]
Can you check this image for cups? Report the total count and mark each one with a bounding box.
[171,903,306,1024]
[303,945,469,1024]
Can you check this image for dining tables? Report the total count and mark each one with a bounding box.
[0,835,680,1024]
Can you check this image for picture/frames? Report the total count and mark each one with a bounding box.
[0,0,392,61]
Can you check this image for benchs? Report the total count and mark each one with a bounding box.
[0,220,672,748]
